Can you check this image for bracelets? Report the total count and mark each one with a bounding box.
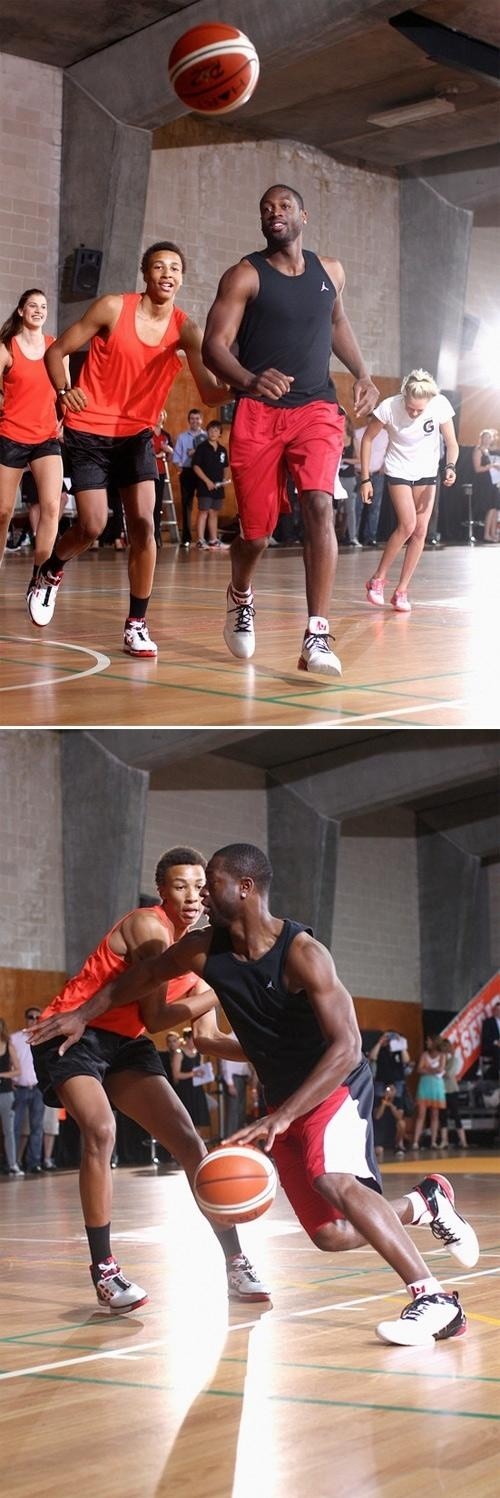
[56,383,70,397]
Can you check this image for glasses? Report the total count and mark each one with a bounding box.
[27,1015,42,1021]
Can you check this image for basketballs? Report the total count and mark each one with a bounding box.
[169,25,258,117]
[192,1142,276,1225]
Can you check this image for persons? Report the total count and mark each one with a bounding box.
[23,408,500,552]
[2,1006,500,1177]
[358,367,459,613]
[199,183,378,678]
[29,849,272,1316]
[21,842,479,1348]
[0,288,71,597]
[25,240,234,659]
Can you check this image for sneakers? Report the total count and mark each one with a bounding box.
[389,588,411,612]
[365,575,385,607]
[394,1140,473,1157]
[224,1251,273,1303]
[410,1172,480,1269]
[25,560,65,629]
[90,541,125,553]
[180,538,232,551]
[347,538,381,549]
[296,617,343,678]
[88,1255,150,1316]
[121,616,159,659]
[8,1158,57,1178]
[222,580,257,661]
[374,1289,469,1347]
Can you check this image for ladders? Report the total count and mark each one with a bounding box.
[156,455,180,544]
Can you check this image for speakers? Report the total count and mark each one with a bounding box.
[60,243,106,304]
[459,309,484,354]
